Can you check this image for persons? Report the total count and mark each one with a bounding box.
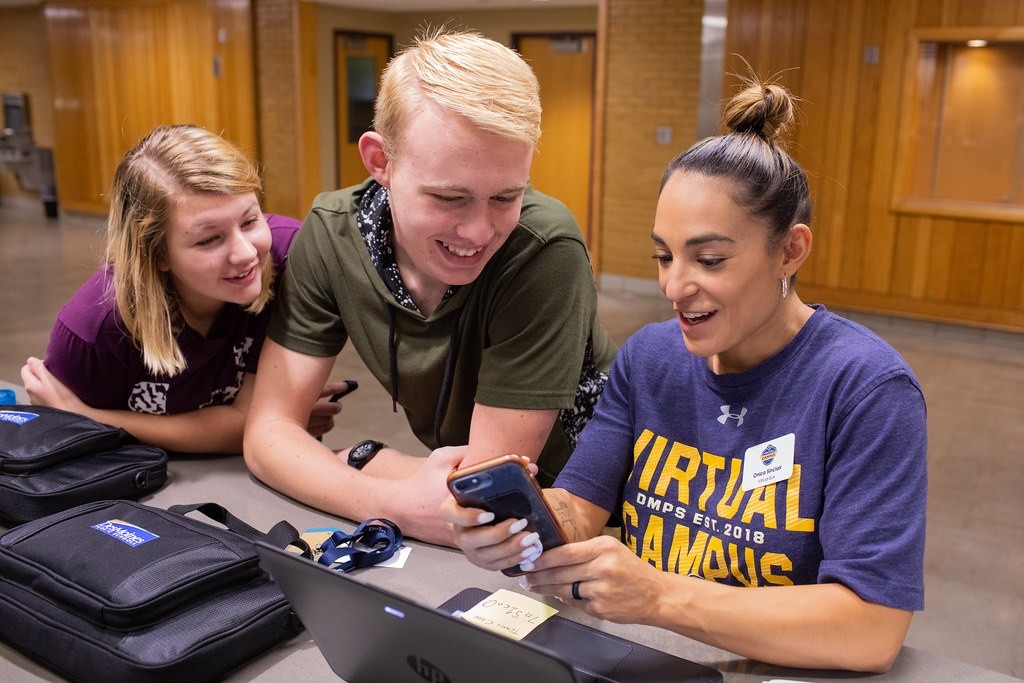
[440,80,929,673]
[242,30,619,551]
[21,126,348,457]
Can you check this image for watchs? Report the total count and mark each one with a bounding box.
[347,439,388,471]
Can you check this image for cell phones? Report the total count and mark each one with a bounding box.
[448,455,572,578]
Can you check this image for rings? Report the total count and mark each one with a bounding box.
[572,582,583,600]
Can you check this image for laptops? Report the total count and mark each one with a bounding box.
[249,540,723,683]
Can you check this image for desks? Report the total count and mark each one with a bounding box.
[0,380,1022,683]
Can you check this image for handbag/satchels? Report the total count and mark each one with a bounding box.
[0,499,315,683]
[1,403,169,529]
